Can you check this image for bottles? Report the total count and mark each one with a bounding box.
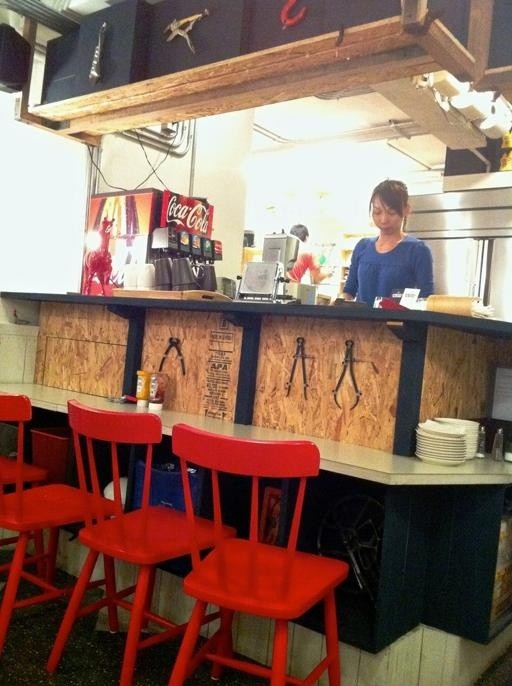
[134,366,170,409]
[476,424,505,462]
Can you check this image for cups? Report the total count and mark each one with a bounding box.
[123,256,218,294]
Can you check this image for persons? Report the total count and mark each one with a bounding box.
[341,177,435,307]
[288,223,338,284]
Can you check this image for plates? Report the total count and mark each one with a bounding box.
[411,413,479,467]
